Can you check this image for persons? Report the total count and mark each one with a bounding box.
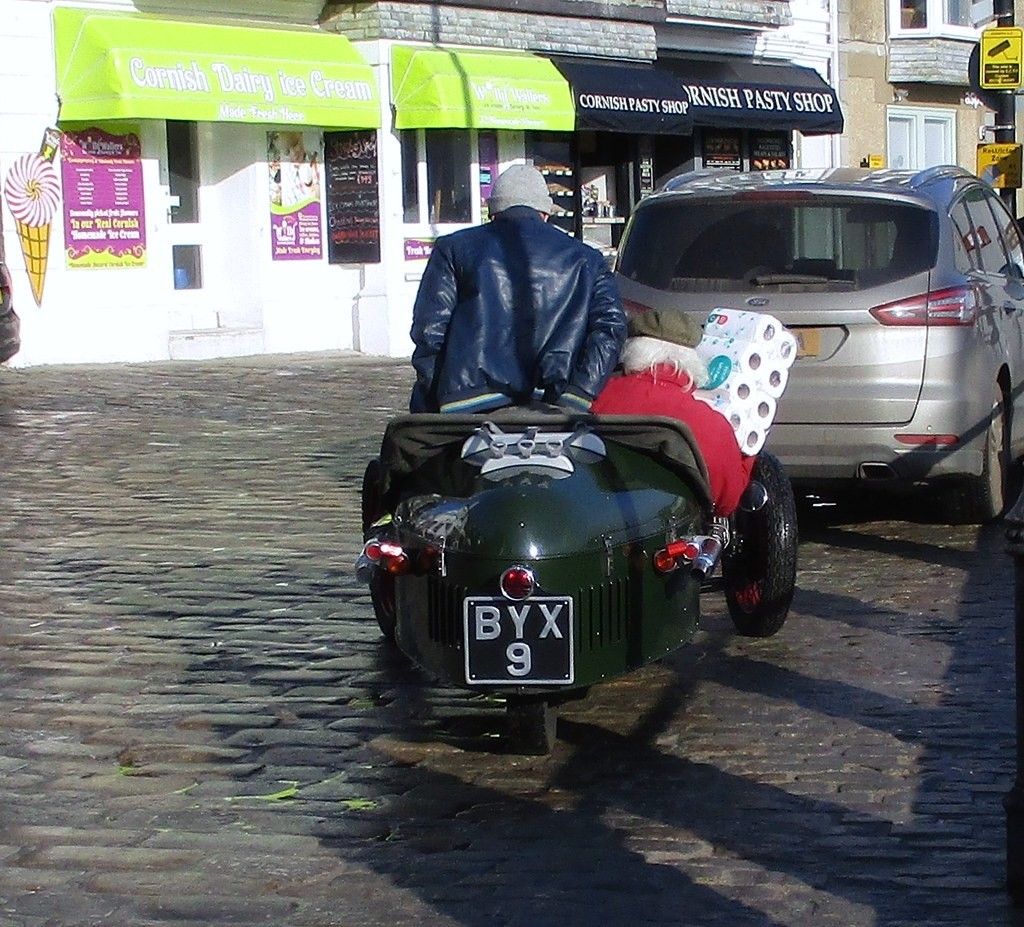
[586,306,758,517]
[267,131,321,210]
[406,162,627,411]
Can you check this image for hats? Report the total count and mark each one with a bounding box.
[627,306,702,348]
[486,164,553,216]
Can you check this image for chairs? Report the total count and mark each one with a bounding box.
[794,258,880,291]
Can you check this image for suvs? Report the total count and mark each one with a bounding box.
[604,163,1024,525]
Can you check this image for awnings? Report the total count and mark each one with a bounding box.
[530,47,695,135]
[389,44,577,133]
[654,47,845,137]
[49,6,381,134]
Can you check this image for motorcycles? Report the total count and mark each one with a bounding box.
[354,389,800,757]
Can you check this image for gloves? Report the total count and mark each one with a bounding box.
[554,385,593,413]
